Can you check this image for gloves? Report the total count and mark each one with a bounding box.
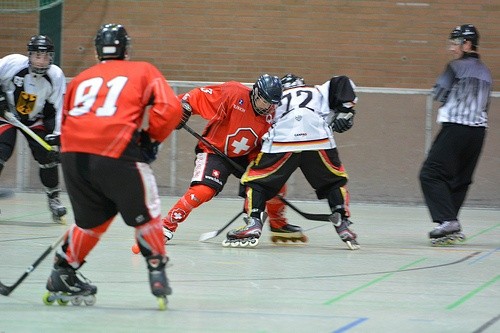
[176,100,193,130]
[328,107,355,133]
[34,135,60,164]
[0,92,10,117]
[138,128,161,164]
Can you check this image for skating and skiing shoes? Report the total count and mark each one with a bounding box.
[428,221,465,247]
[162,226,174,243]
[44,186,67,225]
[222,209,268,247]
[328,213,360,250]
[146,255,172,309]
[270,223,307,243]
[43,251,97,306]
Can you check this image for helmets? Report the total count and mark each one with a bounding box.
[448,24,478,59]
[252,73,282,116]
[27,34,55,77]
[95,24,128,61]
[281,73,305,91]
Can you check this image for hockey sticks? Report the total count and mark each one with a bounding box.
[182,123,331,222]
[200,111,354,241]
[9,117,53,152]
[0,232,68,297]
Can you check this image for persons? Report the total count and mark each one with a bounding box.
[0,33,67,227]
[130,72,308,256]
[417,22,494,247]
[221,72,362,251]
[42,22,182,310]
